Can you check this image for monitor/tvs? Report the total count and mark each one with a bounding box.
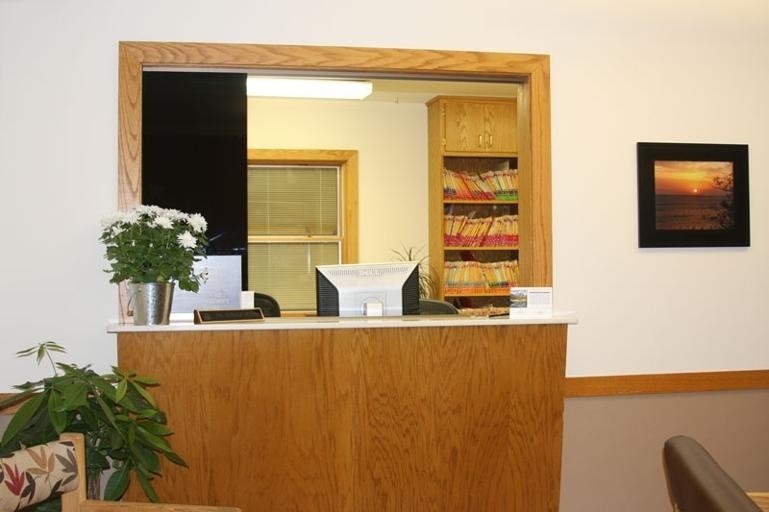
[315,261,419,317]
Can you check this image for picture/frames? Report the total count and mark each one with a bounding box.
[636,142,750,247]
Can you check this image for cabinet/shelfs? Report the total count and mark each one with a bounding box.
[425,96,521,297]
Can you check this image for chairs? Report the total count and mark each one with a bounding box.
[419,298,456,316]
[253,294,281,318]
[663,435,769,511]
[1,433,241,511]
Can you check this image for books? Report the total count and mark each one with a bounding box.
[442,167,520,286]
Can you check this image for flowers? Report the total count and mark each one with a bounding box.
[98,205,208,293]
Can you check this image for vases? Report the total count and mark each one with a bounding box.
[129,281,175,326]
